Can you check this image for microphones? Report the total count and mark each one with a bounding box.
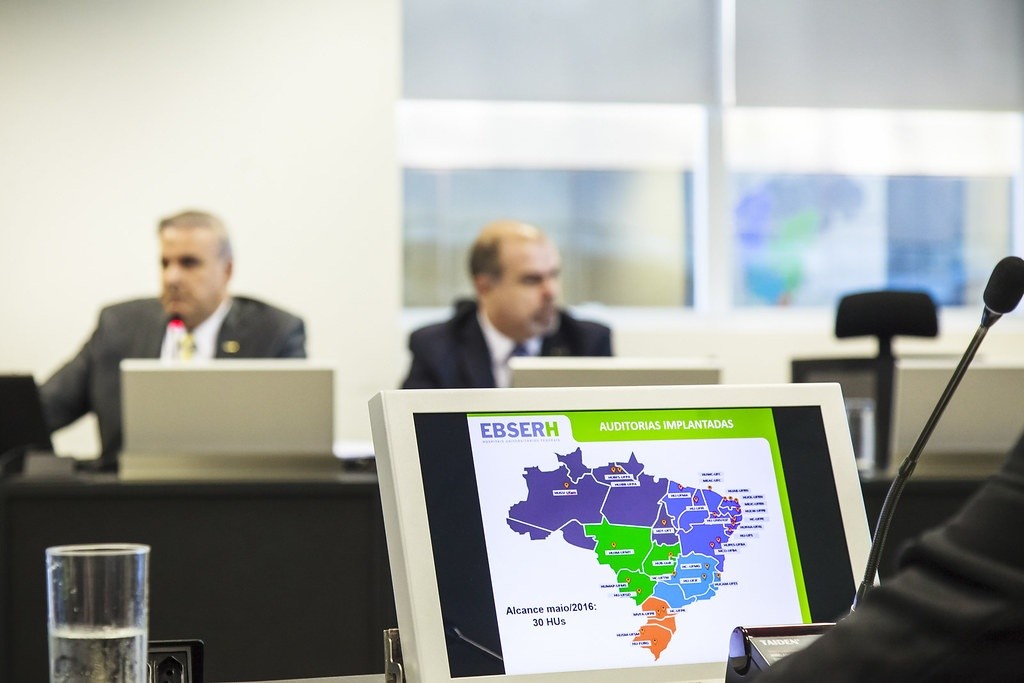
[848,256,1024,615]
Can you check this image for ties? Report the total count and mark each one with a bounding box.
[512,342,530,356]
[179,333,196,361]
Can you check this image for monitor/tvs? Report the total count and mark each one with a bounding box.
[367,381,881,683]
[504,355,724,388]
[115,358,341,485]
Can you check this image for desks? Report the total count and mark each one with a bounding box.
[2,449,1010,682]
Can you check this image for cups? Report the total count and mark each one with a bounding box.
[45,543,151,683]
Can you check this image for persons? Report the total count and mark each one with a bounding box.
[398,221,616,391]
[35,211,308,472]
[745,432,1024,682]
